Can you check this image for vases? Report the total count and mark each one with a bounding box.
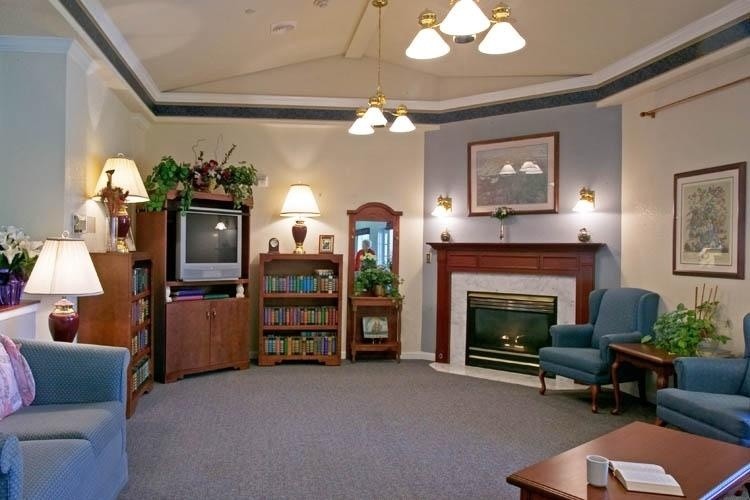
[490,206,518,243]
[0,270,23,305]
[194,177,216,193]
[107,216,118,252]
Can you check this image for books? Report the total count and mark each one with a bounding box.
[132,266,150,295]
[609,459,685,498]
[265,332,337,355]
[132,358,150,395]
[131,297,151,327]
[264,306,338,325]
[132,329,150,356]
[265,268,338,293]
[170,287,229,301]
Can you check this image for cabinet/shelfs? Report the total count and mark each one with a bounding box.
[77,252,155,420]
[136,190,255,287]
[155,297,249,385]
[259,252,343,366]
[345,201,404,363]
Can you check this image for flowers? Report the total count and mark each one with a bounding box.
[1,224,44,270]
[143,132,257,217]
[97,187,130,236]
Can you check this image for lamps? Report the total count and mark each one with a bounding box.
[95,152,151,253]
[24,239,105,343]
[405,0,526,61]
[431,194,454,217]
[572,187,598,213]
[279,184,321,253]
[346,0,417,137]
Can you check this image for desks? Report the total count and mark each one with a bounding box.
[605,343,738,416]
[506,420,750,500]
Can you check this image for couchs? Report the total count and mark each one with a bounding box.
[0,332,131,500]
[538,286,660,413]
[656,313,750,447]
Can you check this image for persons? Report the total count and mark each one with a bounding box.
[354,241,376,272]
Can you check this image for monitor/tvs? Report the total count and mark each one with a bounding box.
[175,205,242,282]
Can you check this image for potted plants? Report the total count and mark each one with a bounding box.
[354,266,405,309]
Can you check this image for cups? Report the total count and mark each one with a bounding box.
[586,454,609,487]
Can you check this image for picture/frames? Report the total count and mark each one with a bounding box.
[362,316,388,338]
[318,234,335,254]
[467,130,560,217]
[672,159,748,281]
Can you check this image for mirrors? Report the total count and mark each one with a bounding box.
[355,220,394,270]
